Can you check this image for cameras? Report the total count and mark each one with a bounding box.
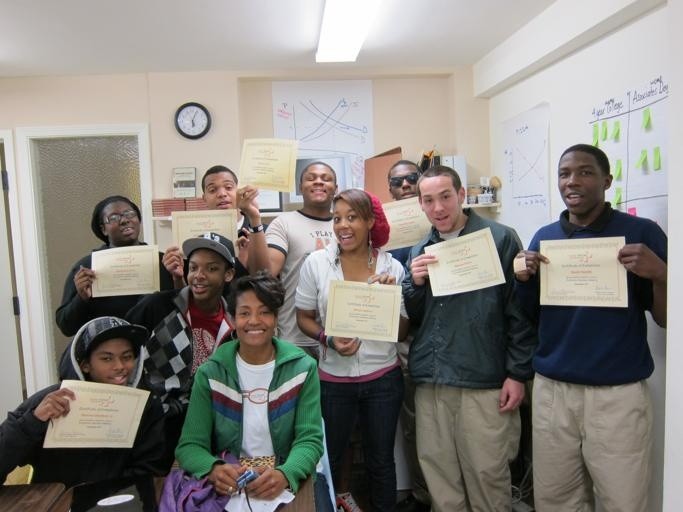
[238,470,260,489]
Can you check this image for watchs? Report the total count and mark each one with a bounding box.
[243,224,265,234]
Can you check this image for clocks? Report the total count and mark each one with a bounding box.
[174,102,211,138]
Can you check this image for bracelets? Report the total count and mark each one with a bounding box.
[318,328,333,360]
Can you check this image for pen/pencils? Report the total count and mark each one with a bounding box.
[480,184,496,194]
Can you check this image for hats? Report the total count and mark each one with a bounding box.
[76,318,149,361]
[184,232,237,264]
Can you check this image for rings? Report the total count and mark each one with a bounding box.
[228,485,233,493]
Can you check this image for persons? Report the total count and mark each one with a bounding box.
[293,187,410,505]
[162,165,270,291]
[144,231,239,418]
[512,143,668,507]
[1,316,175,488]
[400,163,535,507]
[55,195,166,338]
[376,158,428,505]
[235,161,339,377]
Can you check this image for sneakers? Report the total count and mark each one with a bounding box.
[339,491,362,512]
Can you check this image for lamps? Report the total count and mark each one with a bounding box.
[316,0,380,62]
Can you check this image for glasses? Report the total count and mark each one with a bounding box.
[391,172,418,188]
[104,209,137,225]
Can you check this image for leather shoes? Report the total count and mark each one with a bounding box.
[392,493,430,512]
